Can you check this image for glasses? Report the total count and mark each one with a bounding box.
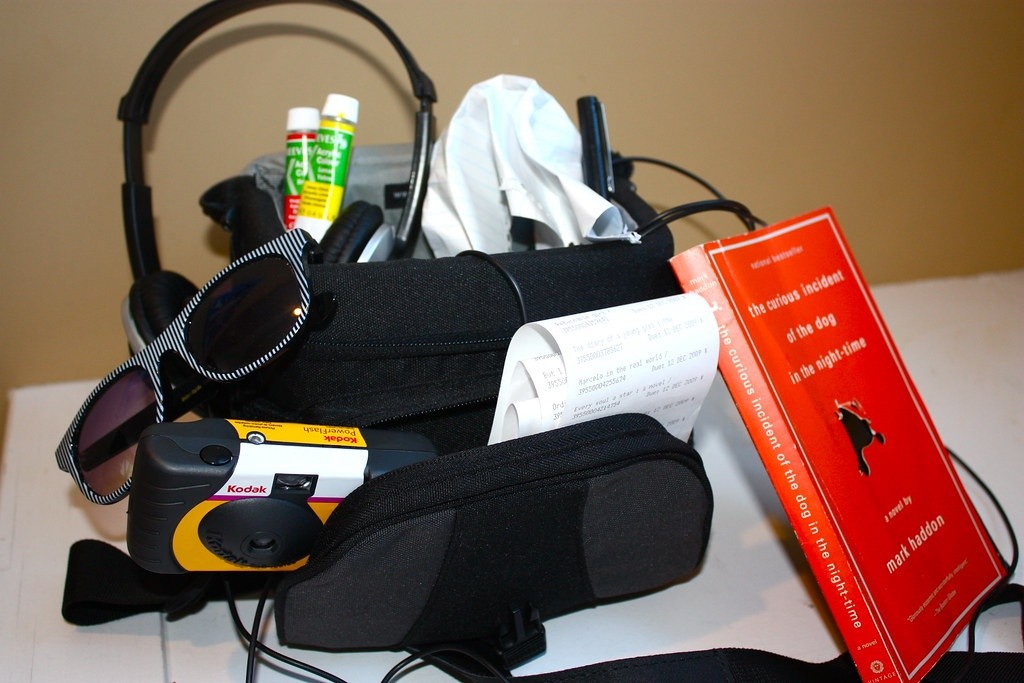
[55,228,324,506]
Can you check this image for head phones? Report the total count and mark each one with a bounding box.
[118,0,437,356]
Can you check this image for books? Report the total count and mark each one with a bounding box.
[669,206,1006,683]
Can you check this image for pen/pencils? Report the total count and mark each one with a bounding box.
[575,95,615,203]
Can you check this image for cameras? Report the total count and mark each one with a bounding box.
[124,416,437,577]
